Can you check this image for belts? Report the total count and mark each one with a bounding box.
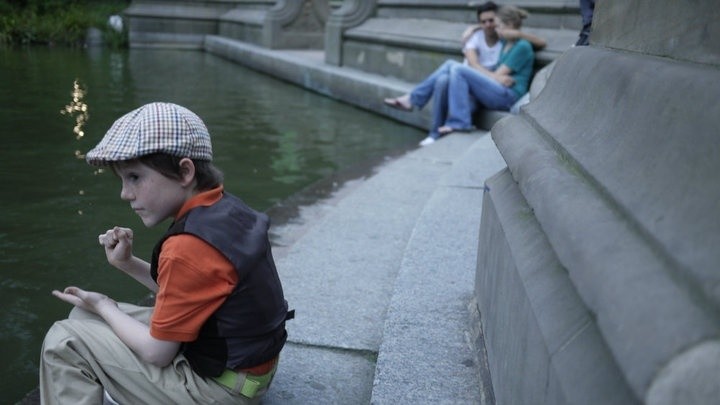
[212,356,278,398]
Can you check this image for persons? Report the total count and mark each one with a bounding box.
[385,2,547,146]
[40,102,295,405]
[439,5,535,132]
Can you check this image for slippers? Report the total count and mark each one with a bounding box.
[384,98,415,111]
[439,126,460,134]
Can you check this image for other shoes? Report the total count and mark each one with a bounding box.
[419,137,434,147]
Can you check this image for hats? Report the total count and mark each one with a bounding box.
[85,102,213,168]
